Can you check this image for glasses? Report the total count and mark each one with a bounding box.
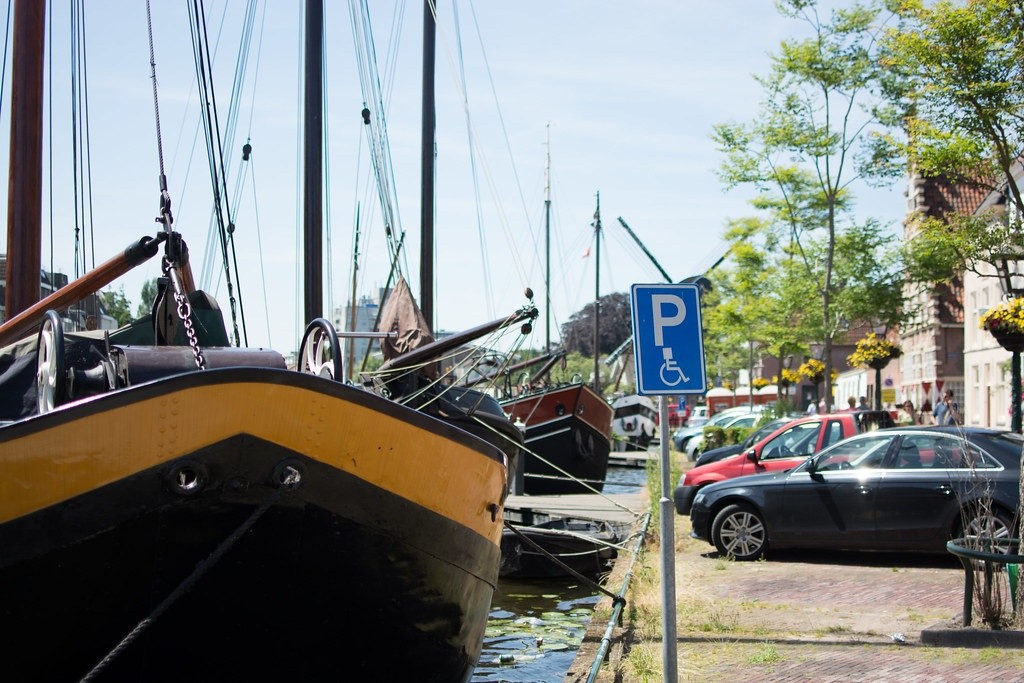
[942,396,950,398]
[905,405,913,407]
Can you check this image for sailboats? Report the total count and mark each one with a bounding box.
[0,1,663,683]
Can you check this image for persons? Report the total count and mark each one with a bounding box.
[847,396,870,411]
[806,397,826,416]
[932,389,960,426]
[897,399,920,427]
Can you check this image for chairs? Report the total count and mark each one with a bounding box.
[901,441,922,467]
[931,439,954,467]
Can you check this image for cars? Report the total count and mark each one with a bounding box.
[666,404,1023,559]
